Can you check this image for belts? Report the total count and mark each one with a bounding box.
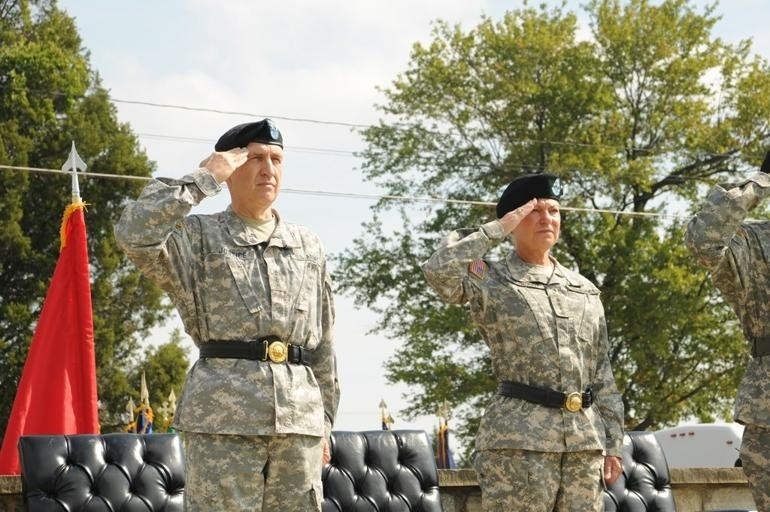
[199,337,315,366]
[497,381,595,413]
[750,334,770,358]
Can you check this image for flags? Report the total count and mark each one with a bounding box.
[123,408,176,435]
[1,201,98,478]
[382,418,388,429]
[437,424,456,469]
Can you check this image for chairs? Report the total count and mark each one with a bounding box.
[319,429,444,512]
[13,427,186,511]
[601,431,675,511]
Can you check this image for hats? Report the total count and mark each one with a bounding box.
[213,119,286,153]
[495,174,564,220]
[760,150,770,173]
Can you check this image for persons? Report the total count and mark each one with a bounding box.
[115,120,339,511]
[422,174,627,512]
[684,152,769,512]
